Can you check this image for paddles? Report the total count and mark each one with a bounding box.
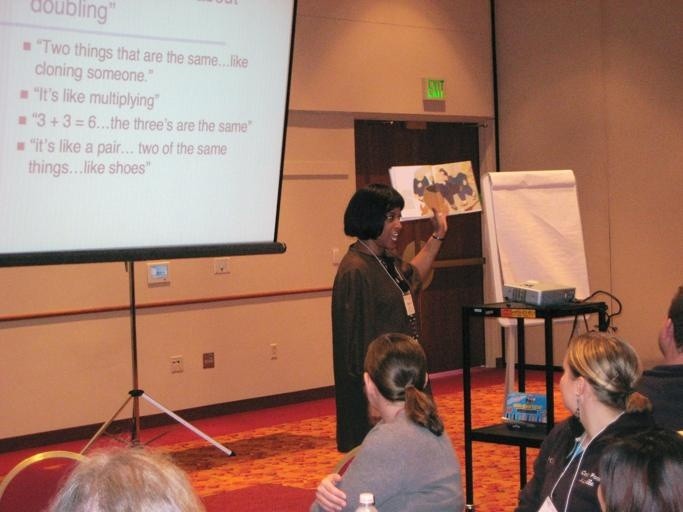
[134,378,575,512]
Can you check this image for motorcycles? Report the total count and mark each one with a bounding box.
[79,259,238,462]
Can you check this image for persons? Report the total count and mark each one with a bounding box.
[513,331,653,512]
[309,333,465,512]
[597,431,682,510]
[331,185,448,453]
[632,287,682,431]
[43,445,206,512]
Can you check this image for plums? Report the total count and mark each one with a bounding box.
[502,279,577,309]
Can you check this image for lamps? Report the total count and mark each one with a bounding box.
[54,449,92,510]
[327,446,366,490]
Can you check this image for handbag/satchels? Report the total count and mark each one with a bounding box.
[430,232,445,243]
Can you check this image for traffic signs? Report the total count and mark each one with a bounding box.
[210,257,234,276]
[203,350,218,369]
[168,352,188,373]
[268,341,283,361]
